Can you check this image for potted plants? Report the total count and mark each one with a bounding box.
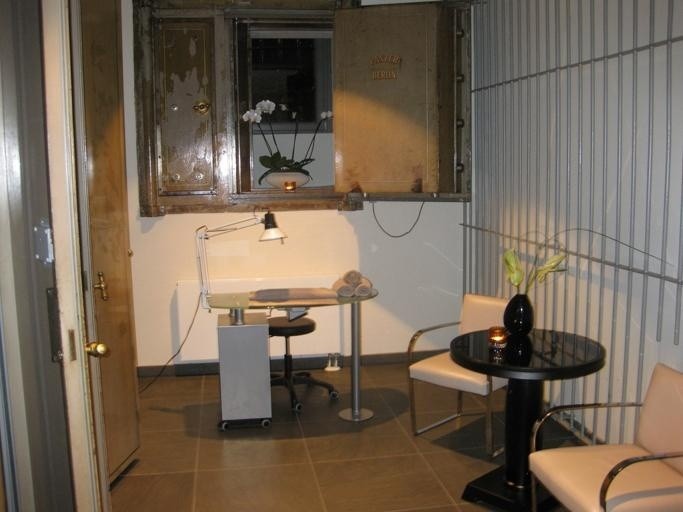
[502,248,568,334]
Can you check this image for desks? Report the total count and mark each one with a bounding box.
[207,289,380,431]
[451,325,607,512]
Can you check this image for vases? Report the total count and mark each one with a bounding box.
[261,171,310,186]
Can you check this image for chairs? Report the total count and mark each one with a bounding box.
[527,362,683,512]
[405,293,537,460]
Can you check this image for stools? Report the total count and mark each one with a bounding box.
[265,316,338,411]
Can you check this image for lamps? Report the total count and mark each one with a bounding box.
[193,206,286,309]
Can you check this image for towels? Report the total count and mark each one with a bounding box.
[332,271,372,297]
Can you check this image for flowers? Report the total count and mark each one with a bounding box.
[240,99,332,185]
